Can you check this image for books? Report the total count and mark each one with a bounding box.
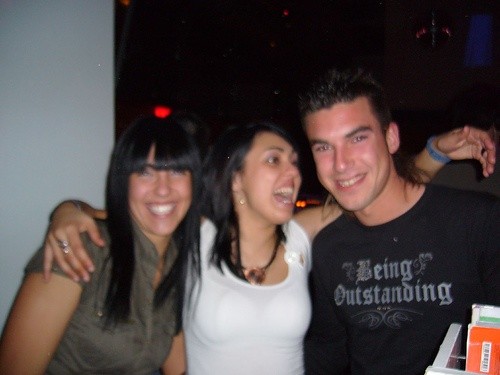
[464,303,500,374]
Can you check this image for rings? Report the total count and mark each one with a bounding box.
[481,148,486,156]
[60,241,71,253]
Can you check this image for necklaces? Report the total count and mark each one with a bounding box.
[228,238,284,285]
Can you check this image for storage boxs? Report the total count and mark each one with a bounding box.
[465,304,500,374]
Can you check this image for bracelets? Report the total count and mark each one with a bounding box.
[425,136,452,164]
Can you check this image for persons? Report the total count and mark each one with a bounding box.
[0,114,202,375]
[42,119,496,375]
[298,65,500,375]
